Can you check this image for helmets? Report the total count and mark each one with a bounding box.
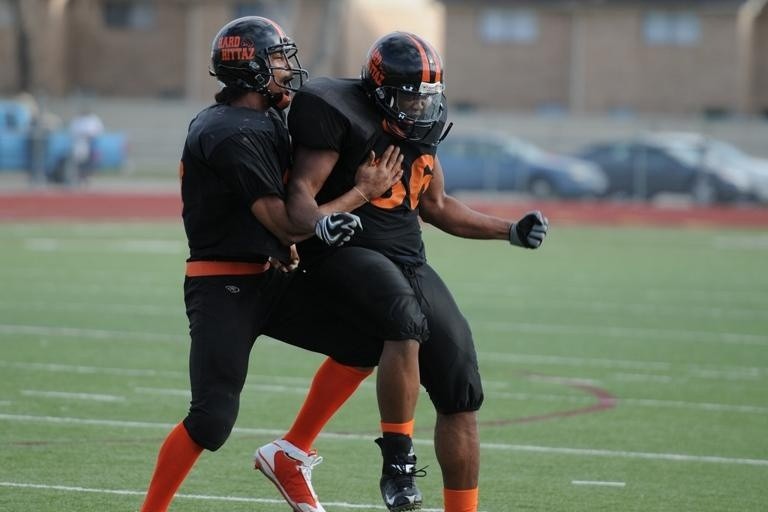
[209,15,310,112]
[359,29,447,143]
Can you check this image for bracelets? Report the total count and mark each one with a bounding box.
[353,187,369,203]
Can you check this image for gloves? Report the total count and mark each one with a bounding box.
[507,209,550,250]
[315,210,365,248]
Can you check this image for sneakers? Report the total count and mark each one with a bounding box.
[252,436,327,512]
[373,435,424,512]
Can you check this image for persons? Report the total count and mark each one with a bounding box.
[283,30,548,512]
[139,15,405,512]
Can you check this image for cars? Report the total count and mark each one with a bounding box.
[585,131,768,204]
[436,133,608,198]
